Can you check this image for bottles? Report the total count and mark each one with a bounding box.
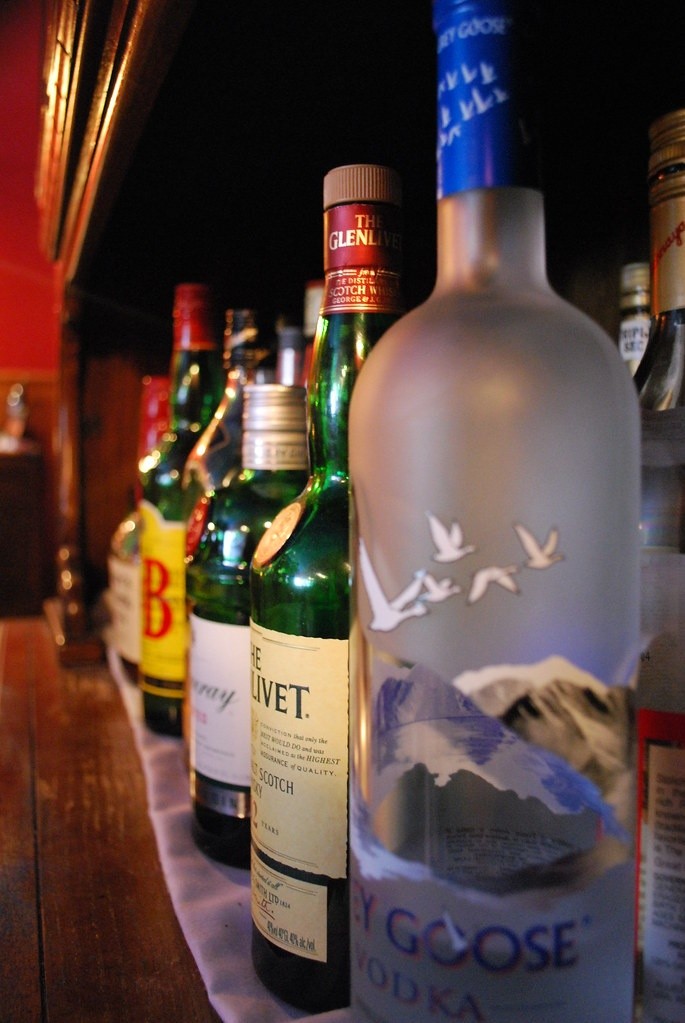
[106,1,685,1022]
